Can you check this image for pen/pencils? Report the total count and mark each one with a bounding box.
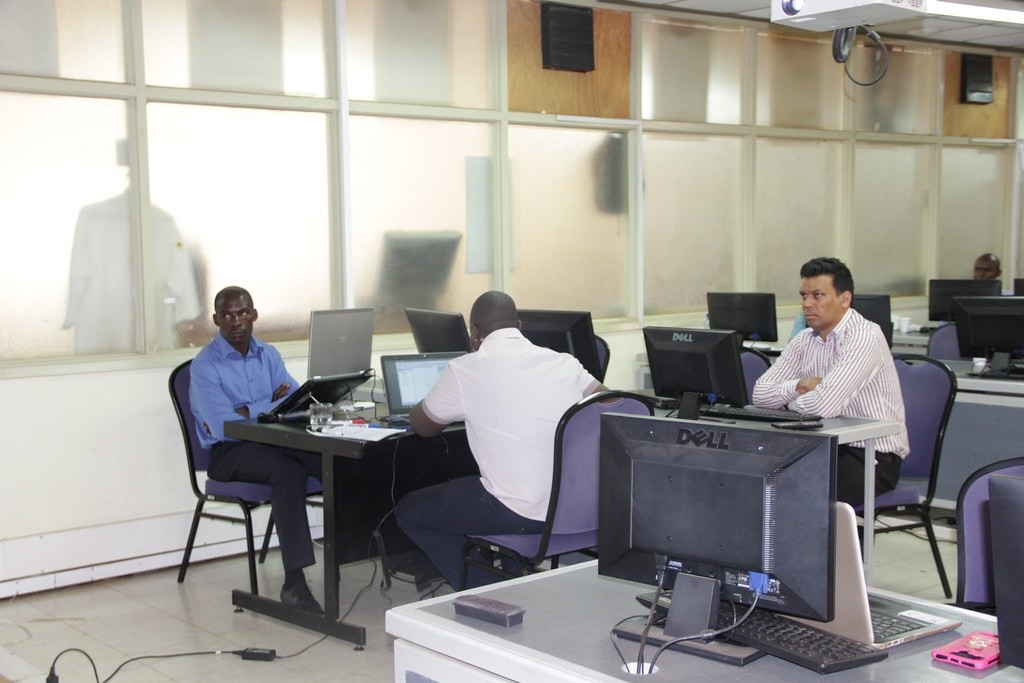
[967,373,978,376]
[344,423,381,428]
[331,419,364,425]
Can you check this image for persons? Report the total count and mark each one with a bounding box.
[973,254,1014,294]
[393,292,616,593]
[189,287,323,612]
[751,257,908,508]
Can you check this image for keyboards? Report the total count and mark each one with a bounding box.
[699,402,823,423]
[633,588,889,675]
[760,349,783,356]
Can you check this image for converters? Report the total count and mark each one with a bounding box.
[242,647,276,661]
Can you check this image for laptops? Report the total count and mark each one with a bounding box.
[405,308,471,355]
[270,308,376,414]
[987,474,1024,671]
[777,501,964,649]
[380,351,469,424]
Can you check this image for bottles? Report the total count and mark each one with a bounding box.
[703,313,710,329]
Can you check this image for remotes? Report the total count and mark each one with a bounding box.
[771,422,824,430]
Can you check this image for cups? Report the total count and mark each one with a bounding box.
[898,317,911,334]
[973,358,987,367]
[310,402,333,432]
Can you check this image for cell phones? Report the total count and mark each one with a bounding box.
[930,630,1000,670]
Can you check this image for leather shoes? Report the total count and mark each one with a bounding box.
[280,585,324,614]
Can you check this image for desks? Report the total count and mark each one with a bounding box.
[652,405,908,585]
[893,329,930,345]
[383,555,1024,683]
[901,357,1024,542]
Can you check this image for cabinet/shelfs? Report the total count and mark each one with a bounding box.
[223,407,474,652]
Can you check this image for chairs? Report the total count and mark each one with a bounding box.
[595,334,611,381]
[853,353,961,598]
[927,325,971,361]
[955,457,1024,616]
[167,357,322,596]
[463,391,656,591]
[739,345,774,403]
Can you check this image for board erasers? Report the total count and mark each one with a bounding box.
[453,595,525,627]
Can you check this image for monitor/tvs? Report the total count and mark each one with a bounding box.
[928,278,1024,370]
[852,294,892,352]
[516,309,603,386]
[708,292,778,344]
[596,412,839,667]
[643,325,750,423]
